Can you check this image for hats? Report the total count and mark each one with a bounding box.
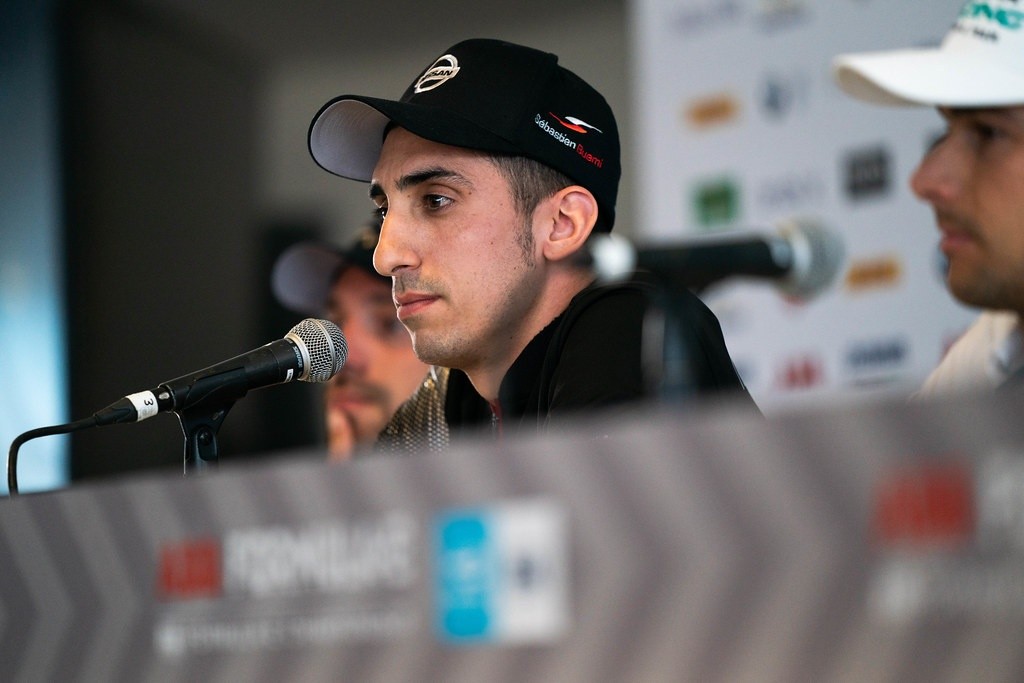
[831,1,1023,107]
[270,224,391,314]
[306,39,622,236]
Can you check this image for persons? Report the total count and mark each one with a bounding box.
[306,37,773,459]
[270,220,436,461]
[831,0,1024,401]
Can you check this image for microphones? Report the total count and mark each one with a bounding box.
[584,216,847,302]
[95,318,350,424]
